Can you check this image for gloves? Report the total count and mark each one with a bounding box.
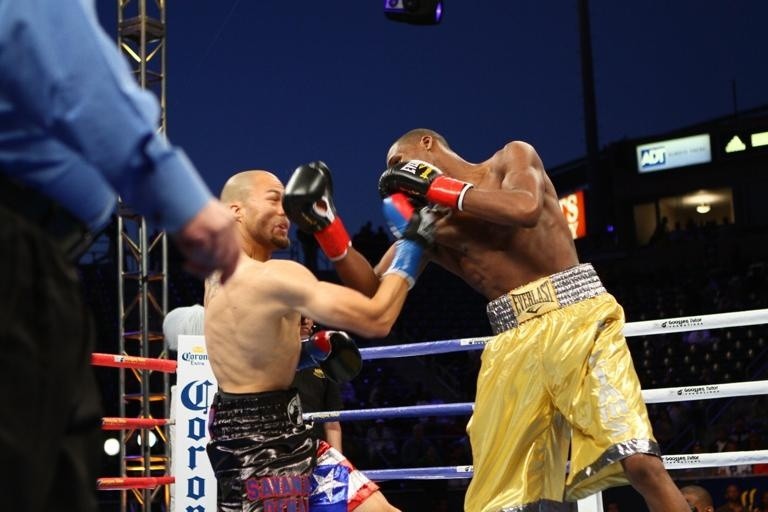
[382,194,451,289]
[295,331,362,384]
[282,162,353,262]
[379,160,472,211]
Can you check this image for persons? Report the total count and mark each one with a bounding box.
[91,167,768,510]
[0,0,247,511]
[279,125,695,512]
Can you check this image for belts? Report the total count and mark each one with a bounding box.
[0,172,94,259]
